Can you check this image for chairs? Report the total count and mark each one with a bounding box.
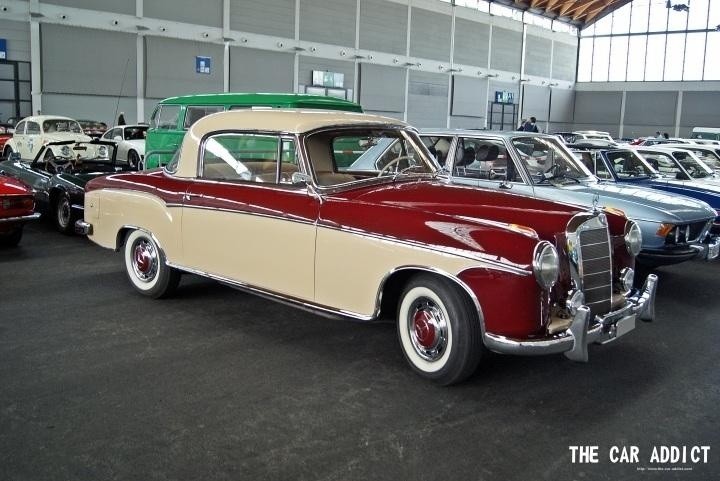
[455,145,501,179]
[306,141,356,185]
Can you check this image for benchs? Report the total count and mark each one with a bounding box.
[203,162,298,181]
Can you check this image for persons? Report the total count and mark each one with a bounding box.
[664,133,670,139]
[523,117,538,133]
[656,132,662,138]
[517,121,526,131]
